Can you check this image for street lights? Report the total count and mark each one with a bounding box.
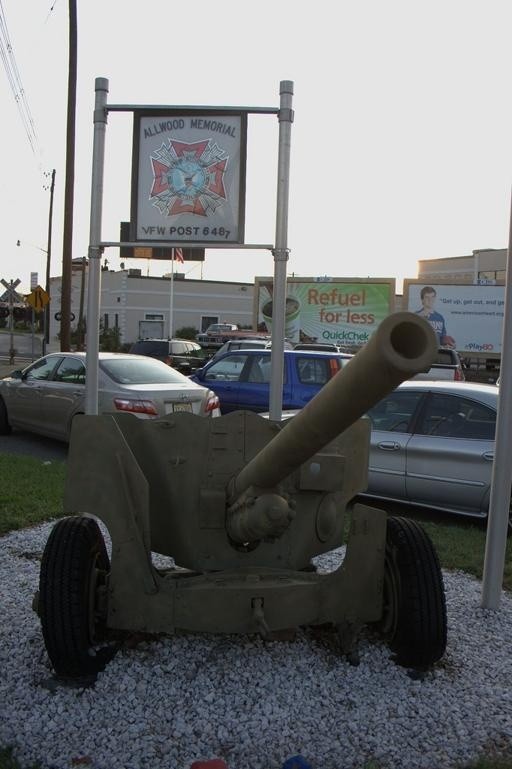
[16,238,51,345]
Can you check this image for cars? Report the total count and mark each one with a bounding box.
[245,378,511,537]
[0,347,223,453]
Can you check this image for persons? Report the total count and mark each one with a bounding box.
[414,287,446,345]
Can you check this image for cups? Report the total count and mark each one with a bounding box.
[264,296,301,343]
